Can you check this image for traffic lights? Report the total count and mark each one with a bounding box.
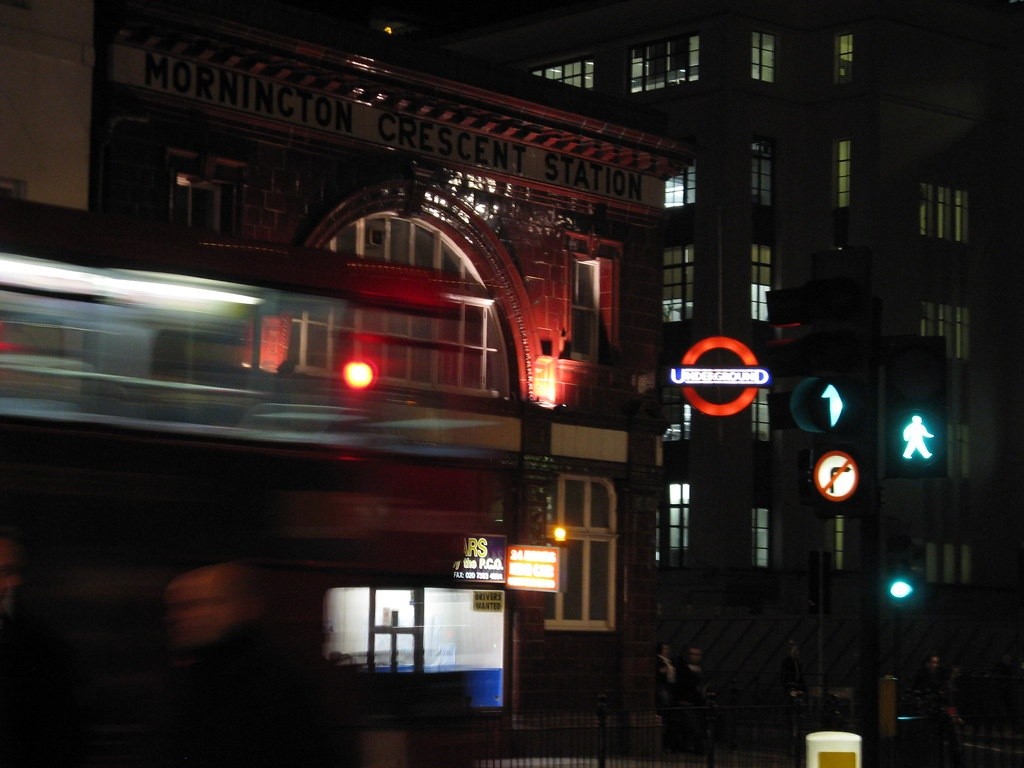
[881,333,948,479]
[787,369,864,445]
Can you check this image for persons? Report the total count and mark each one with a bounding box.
[0,527,72,768]
[157,563,362,768]
[656,640,1021,756]
